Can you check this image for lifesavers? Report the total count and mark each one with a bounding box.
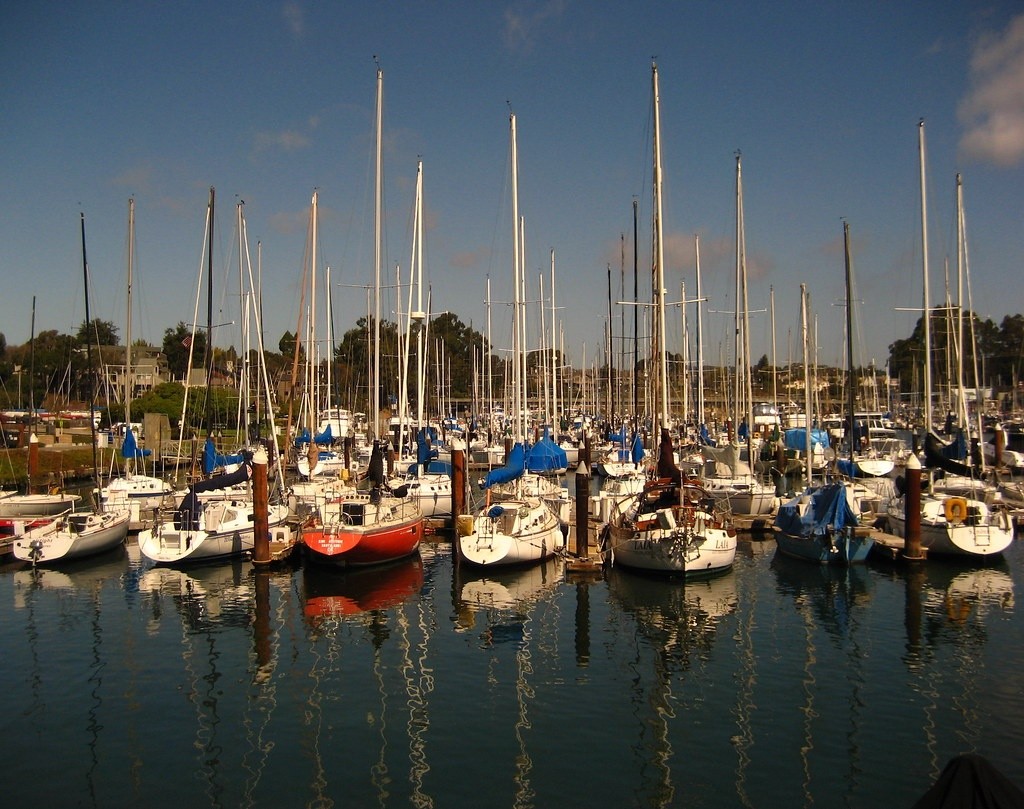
[945,497,967,524]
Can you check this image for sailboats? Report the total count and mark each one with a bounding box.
[1,53,1024,574]
